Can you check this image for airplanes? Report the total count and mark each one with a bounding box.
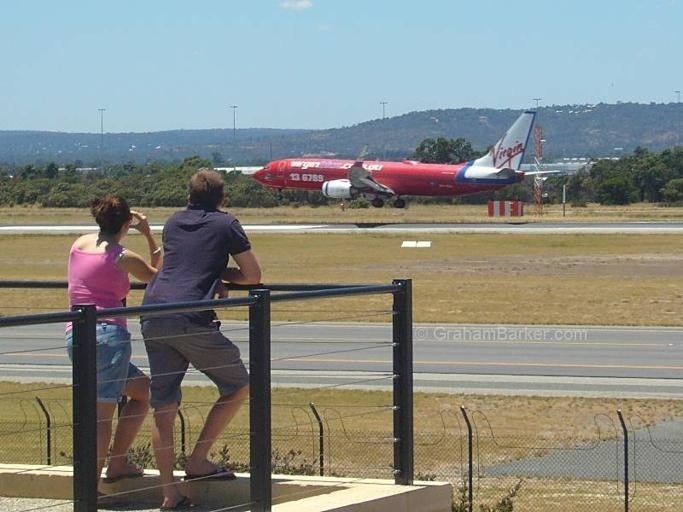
[246,111,565,209]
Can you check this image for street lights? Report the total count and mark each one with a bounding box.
[379,102,389,118]
[229,105,239,137]
[532,98,544,108]
[96,109,107,146]
[673,90,680,103]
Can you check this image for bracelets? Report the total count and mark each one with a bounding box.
[147,244,162,258]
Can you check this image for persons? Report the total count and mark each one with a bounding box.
[67,190,161,479]
[139,170,261,510]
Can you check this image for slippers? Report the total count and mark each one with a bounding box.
[102,459,144,483]
[97,491,121,508]
[160,495,201,511]
[181,462,236,483]
